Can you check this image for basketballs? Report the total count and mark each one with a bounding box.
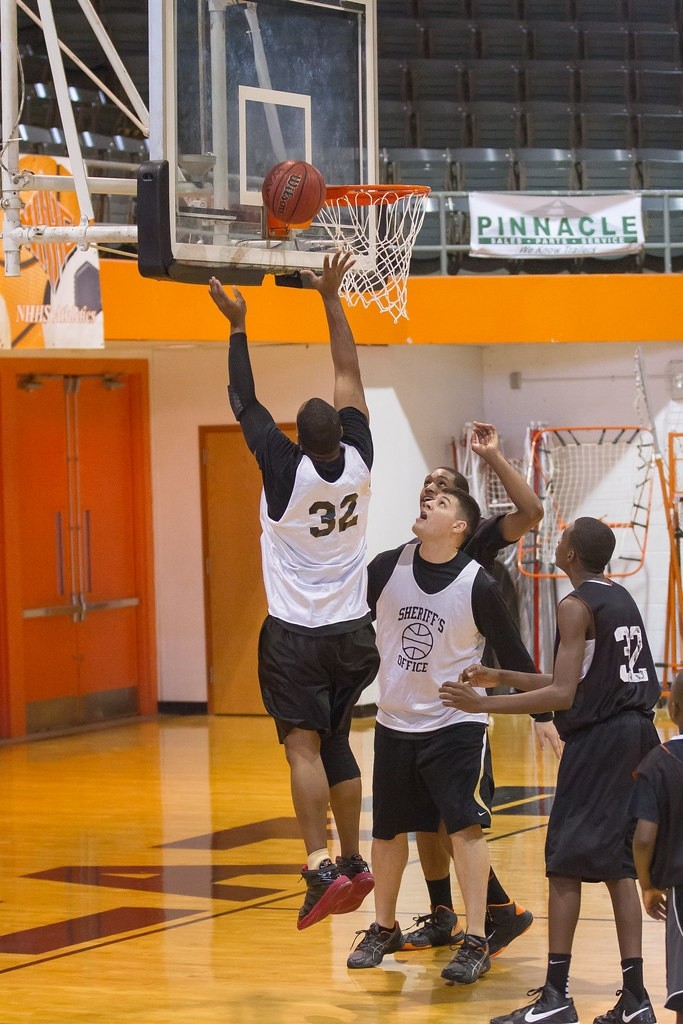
[260,158,327,226]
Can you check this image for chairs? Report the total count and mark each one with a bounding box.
[177,1,357,231]
[15,0,150,256]
[376,0,683,275]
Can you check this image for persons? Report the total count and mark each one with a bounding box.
[438,517,683,1024]
[347,420,563,983]
[208,251,382,930]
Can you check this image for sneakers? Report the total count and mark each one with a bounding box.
[485,898,534,958]
[400,905,465,951]
[297,854,375,931]
[593,986,657,1024]
[489,984,581,1024]
[347,919,406,968]
[441,934,491,984]
